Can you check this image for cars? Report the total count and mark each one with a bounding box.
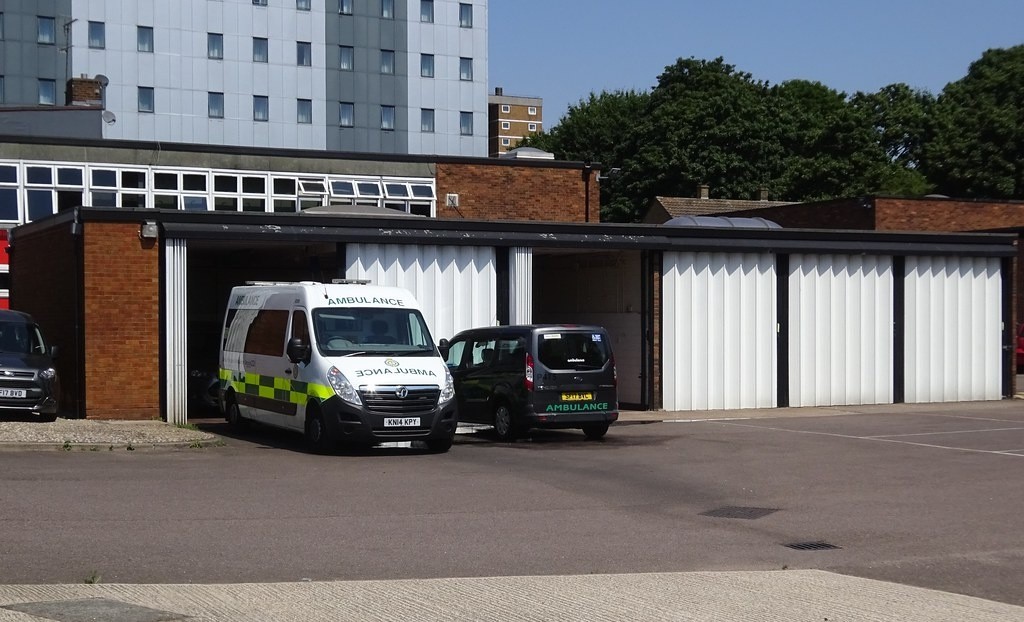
[1,309,60,422]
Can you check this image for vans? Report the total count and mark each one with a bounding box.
[437,324,619,439]
[218,279,456,454]
